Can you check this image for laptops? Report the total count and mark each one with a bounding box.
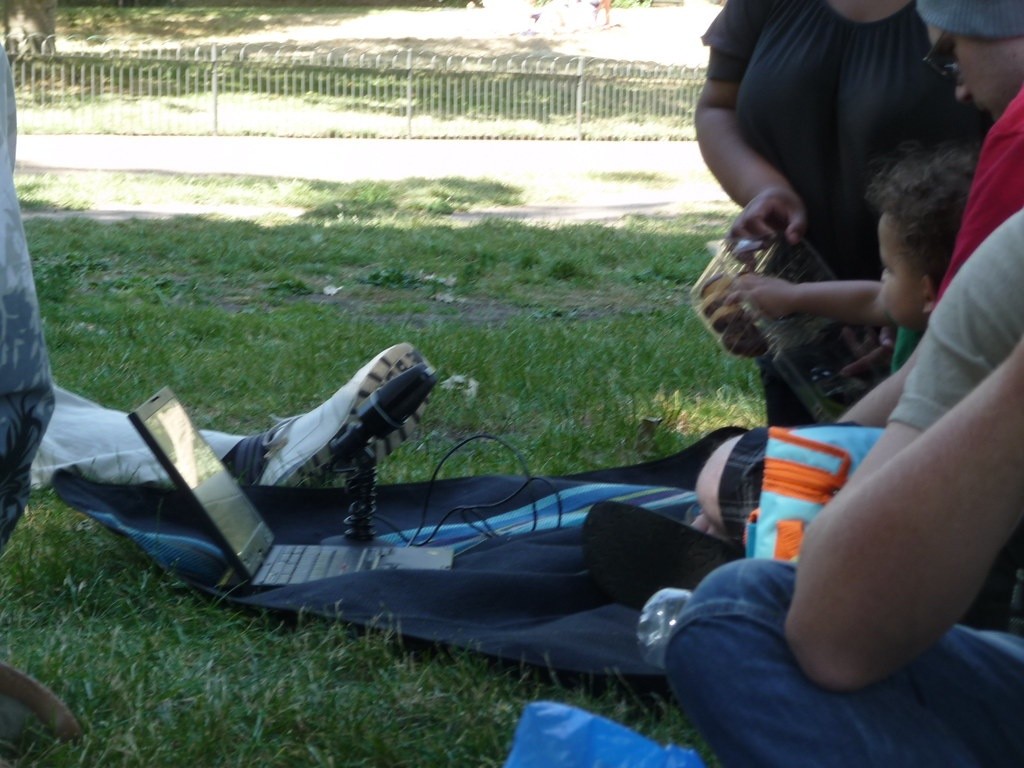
[127,385,454,594]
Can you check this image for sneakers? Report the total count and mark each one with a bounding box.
[258,340,435,489]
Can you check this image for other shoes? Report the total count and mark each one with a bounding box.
[582,500,746,614]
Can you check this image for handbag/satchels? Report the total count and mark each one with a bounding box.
[742,425,889,564]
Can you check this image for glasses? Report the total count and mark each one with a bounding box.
[923,29,956,77]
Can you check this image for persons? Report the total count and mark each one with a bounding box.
[687,0,1024,537]
[0,42,56,548]
[663,206,1024,768]
[29,341,438,495]
[589,0,615,26]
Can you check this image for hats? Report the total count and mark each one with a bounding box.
[917,0,1023,39]
